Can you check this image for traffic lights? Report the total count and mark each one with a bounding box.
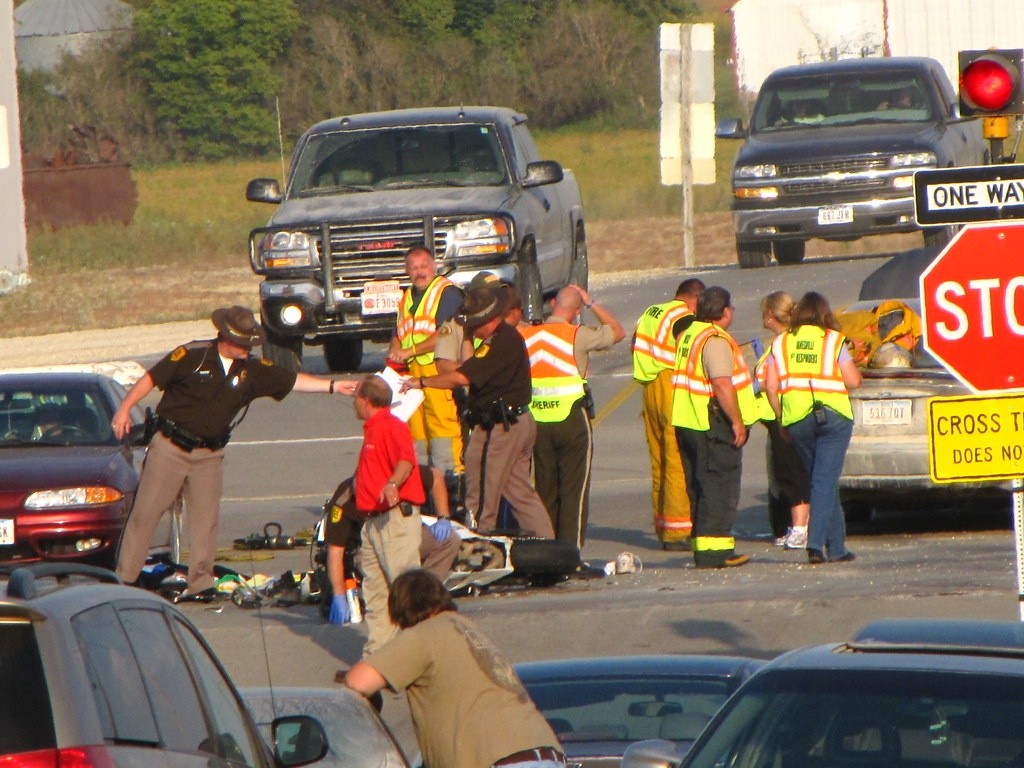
[957,48,1024,116]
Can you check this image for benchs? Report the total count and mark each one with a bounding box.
[838,736,1024,768]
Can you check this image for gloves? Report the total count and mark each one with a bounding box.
[330,594,350,625]
[429,519,452,540]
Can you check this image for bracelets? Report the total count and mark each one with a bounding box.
[438,516,450,521]
[388,480,399,487]
[412,344,416,354]
[587,300,595,308]
[421,376,427,386]
[329,380,334,393]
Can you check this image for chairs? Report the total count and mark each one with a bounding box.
[850,725,966,768]
[338,167,377,186]
[657,711,712,740]
[545,717,574,734]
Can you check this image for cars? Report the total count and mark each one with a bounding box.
[0,372,183,579]
[617,615,1023,768]
[513,654,767,768]
[835,298,1018,537]
[230,677,413,768]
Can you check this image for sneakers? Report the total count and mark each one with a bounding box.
[785,525,808,549]
[773,527,793,546]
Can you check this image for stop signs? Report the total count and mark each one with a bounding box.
[920,223,1024,395]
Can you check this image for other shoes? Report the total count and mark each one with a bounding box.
[827,551,856,562]
[190,587,229,603]
[663,536,695,552]
[320,590,333,619]
[807,548,829,564]
[334,670,351,682]
[617,553,644,573]
[568,561,606,581]
[693,549,750,568]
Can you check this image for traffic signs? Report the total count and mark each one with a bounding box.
[912,162,1024,228]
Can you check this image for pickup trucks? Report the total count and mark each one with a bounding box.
[245,105,591,373]
[714,56,992,270]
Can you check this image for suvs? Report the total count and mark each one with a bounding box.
[0,560,331,767]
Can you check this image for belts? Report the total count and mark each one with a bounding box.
[515,406,530,415]
[493,746,568,766]
[197,440,213,451]
[365,506,402,519]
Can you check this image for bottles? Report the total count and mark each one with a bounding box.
[345,579,362,623]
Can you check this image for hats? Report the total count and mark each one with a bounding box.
[471,271,509,289]
[211,305,267,347]
[455,286,509,326]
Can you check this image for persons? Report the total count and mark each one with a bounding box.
[323,467,463,625]
[519,283,627,551]
[345,568,567,768]
[351,376,426,658]
[875,76,916,111]
[670,285,761,570]
[111,307,367,601]
[630,278,707,552]
[752,291,862,564]
[386,246,557,539]
[775,88,808,125]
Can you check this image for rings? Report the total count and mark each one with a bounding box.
[113,426,116,427]
[395,498,396,500]
